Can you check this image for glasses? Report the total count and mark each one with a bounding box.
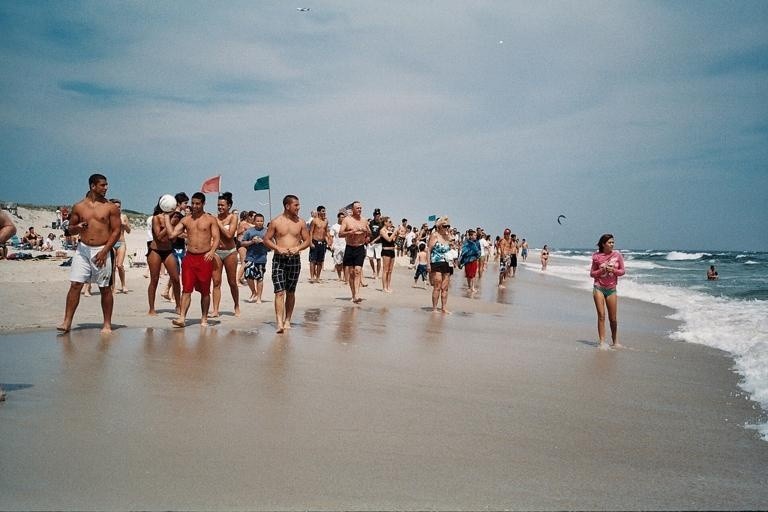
[442,225,451,229]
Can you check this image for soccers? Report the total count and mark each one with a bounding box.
[159,194,177,213]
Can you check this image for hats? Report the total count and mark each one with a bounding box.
[504,230,511,235]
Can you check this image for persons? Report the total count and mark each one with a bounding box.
[80,282,93,298]
[262,194,311,335]
[0,209,17,243]
[142,188,274,329]
[539,244,549,271]
[307,200,529,317]
[108,197,130,294]
[55,172,122,335]
[42,233,54,251]
[706,264,719,281]
[589,233,625,348]
[55,206,61,229]
[23,226,40,251]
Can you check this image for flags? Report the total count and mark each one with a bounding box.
[252,176,269,190]
[200,176,219,193]
